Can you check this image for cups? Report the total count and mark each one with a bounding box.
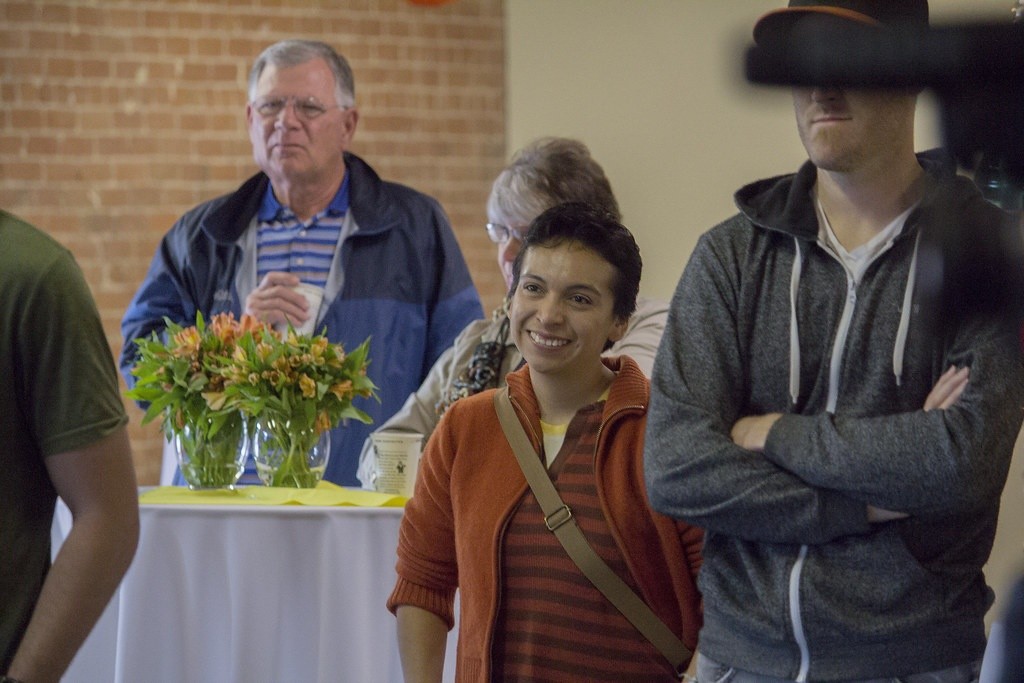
[371,432,423,497]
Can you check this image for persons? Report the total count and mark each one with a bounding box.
[0,209,141,683]
[355,0,1024,683]
[121,41,485,488]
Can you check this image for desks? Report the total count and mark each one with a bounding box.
[50,484,459,683]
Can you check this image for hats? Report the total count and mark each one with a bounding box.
[753,0,929,45]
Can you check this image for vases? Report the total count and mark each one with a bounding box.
[250,414,332,489]
[175,408,251,490]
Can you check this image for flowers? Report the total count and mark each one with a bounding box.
[203,312,382,490]
[117,307,284,485]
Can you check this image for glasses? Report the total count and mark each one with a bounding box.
[485,223,529,244]
[249,95,348,122]
